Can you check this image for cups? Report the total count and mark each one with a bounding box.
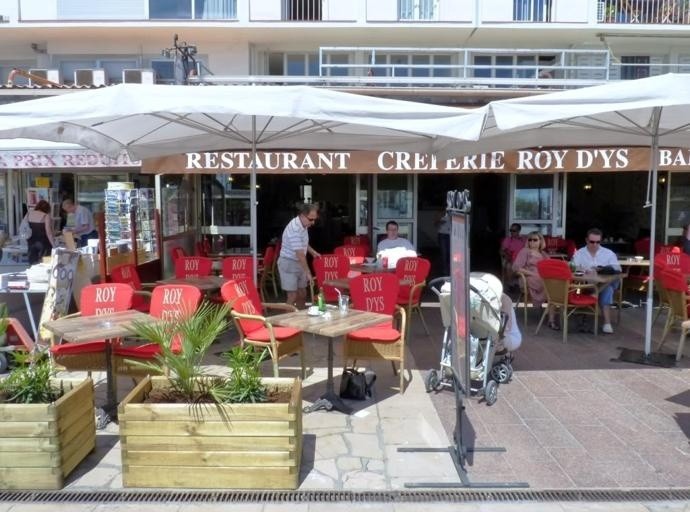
[338,295,349,316]
[309,306,319,315]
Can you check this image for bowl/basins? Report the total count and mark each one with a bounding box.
[366,257,375,263]
[635,257,644,262]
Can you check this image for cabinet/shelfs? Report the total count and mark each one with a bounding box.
[25,186,61,233]
[138,188,156,252]
[103,181,139,250]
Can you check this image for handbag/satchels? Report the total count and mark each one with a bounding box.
[18,211,32,242]
[338,367,372,400]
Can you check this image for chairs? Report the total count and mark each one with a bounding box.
[656,270,690,361]
[343,235,370,255]
[113,285,203,376]
[171,246,188,264]
[111,262,155,311]
[340,274,407,394]
[49,283,134,380]
[511,271,530,331]
[396,256,431,344]
[534,258,600,344]
[221,277,306,380]
[193,240,202,256]
[271,242,281,298]
[204,236,211,254]
[310,254,350,303]
[223,256,252,279]
[335,244,368,277]
[175,256,212,277]
[254,246,274,311]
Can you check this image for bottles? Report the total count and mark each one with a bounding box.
[569,256,576,273]
[317,286,325,311]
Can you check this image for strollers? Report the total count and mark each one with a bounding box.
[425,271,522,405]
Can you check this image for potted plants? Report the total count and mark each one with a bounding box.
[118,296,304,491]
[0,345,97,490]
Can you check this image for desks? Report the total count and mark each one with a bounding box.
[0,244,98,343]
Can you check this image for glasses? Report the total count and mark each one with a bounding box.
[303,214,319,221]
[509,229,519,233]
[527,238,541,243]
[587,238,601,245]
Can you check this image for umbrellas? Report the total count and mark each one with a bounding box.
[482,70,690,354]
[0,82,487,290]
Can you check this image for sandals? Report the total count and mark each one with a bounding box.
[547,320,560,331]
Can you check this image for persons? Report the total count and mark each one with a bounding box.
[375,220,417,269]
[62,199,99,247]
[511,232,560,330]
[25,200,57,266]
[277,204,318,309]
[571,229,622,334]
[675,224,690,254]
[499,223,526,269]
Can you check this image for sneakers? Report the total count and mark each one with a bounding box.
[602,323,613,334]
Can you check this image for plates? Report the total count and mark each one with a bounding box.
[306,311,323,316]
